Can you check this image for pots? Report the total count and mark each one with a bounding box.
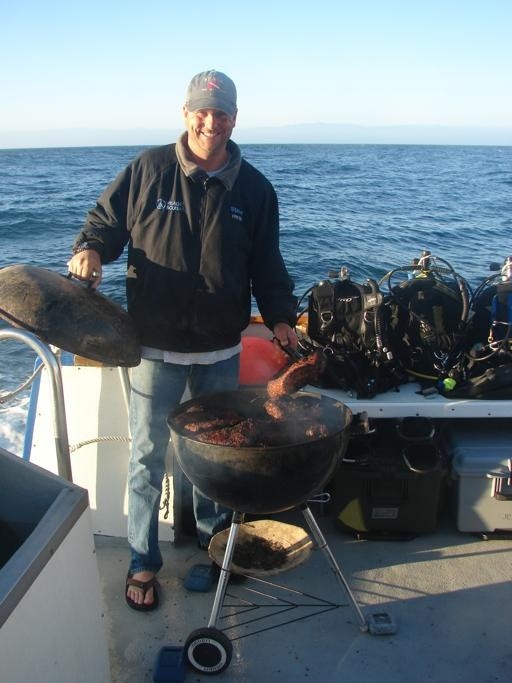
[165,388,352,514]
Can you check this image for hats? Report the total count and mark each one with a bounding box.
[186,69,236,117]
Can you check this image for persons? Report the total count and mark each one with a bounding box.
[68,68,300,612]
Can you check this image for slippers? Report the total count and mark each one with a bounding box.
[126,571,158,611]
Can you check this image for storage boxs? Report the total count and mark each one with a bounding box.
[329,418,511,540]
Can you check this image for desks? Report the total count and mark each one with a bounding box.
[295,376,511,425]
